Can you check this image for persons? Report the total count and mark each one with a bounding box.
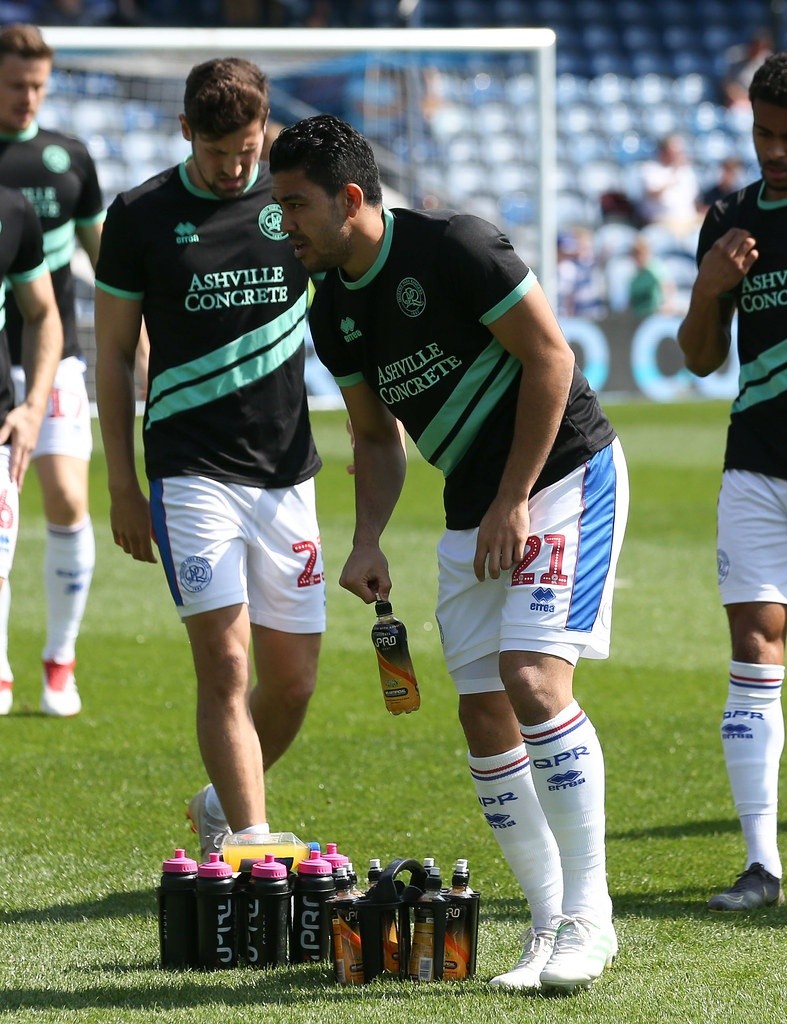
[556,27,776,330]
[678,54,787,912]
[0,24,150,716]
[94,57,327,860]
[268,113,630,989]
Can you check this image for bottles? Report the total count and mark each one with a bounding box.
[371,601,421,715]
[159,844,480,987]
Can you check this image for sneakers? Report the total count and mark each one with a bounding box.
[41,647,81,717]
[540,905,619,985]
[707,861,785,913]
[489,926,557,989]
[187,783,234,866]
[0,677,14,715]
